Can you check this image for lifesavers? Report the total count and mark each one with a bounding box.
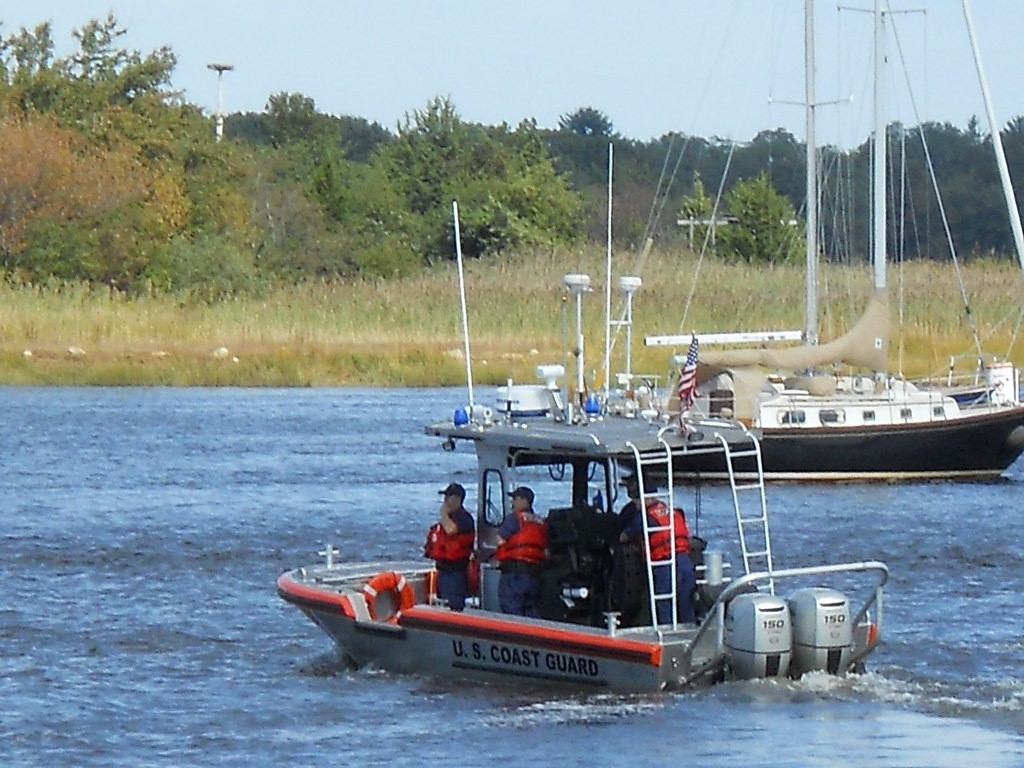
[363,573,415,626]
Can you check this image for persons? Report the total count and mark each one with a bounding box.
[612,472,648,528]
[497,487,548,618]
[618,479,697,621]
[425,484,474,611]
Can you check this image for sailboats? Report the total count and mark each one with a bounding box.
[479,0,1022,482]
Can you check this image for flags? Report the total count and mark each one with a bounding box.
[677,338,697,437]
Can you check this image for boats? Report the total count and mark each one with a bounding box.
[275,133,887,695]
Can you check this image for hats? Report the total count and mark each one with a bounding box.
[507,487,533,499]
[619,471,658,494]
[438,484,464,495]
